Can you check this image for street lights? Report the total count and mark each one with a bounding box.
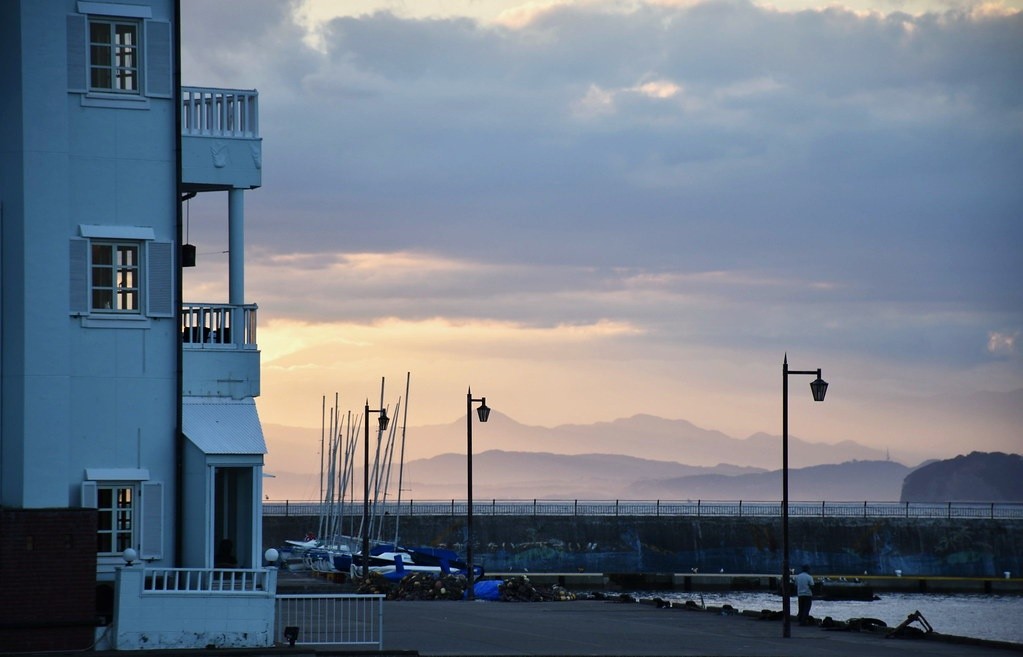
[780,352,831,640]
[467,388,492,570]
[361,405,392,580]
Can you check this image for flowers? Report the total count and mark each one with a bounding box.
[497,575,578,602]
[353,569,466,601]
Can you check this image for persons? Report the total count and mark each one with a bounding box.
[793,564,814,625]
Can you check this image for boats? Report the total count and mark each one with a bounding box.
[285,534,483,584]
